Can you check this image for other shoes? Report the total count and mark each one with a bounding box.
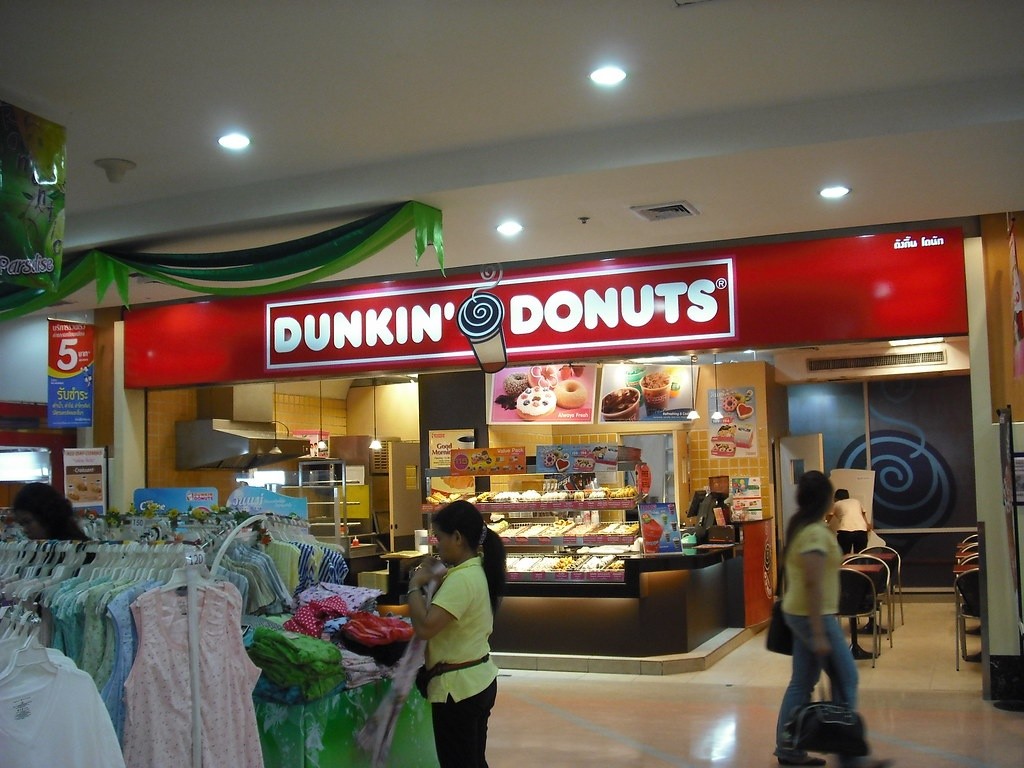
[778,755,826,766]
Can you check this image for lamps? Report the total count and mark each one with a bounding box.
[369,378,382,449]
[268,382,282,454]
[305,380,316,448]
[687,357,700,419]
[712,354,723,419]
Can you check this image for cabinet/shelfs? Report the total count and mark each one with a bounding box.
[423,465,650,588]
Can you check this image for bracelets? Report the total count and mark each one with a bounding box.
[408,588,419,594]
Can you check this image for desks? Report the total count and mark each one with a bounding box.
[951,562,982,662]
[955,550,980,564]
[843,551,895,635]
[839,563,885,659]
[956,541,978,550]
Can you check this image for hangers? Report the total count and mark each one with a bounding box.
[0,496,320,696]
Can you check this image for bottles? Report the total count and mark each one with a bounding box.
[353,536,359,546]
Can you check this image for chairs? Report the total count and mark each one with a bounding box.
[836,528,981,671]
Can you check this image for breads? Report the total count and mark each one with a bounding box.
[599,524,640,534]
[425,486,623,568]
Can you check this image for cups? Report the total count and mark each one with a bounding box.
[639,375,671,416]
[601,386,640,421]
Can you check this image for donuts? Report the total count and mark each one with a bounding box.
[721,393,745,412]
[543,449,562,467]
[610,486,637,498]
[575,459,593,468]
[504,365,586,421]
[712,443,735,452]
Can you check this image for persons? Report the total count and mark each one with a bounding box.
[408,500,505,768]
[773,470,894,768]
[826,489,871,553]
[13,484,97,575]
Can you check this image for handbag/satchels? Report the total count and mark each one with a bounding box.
[415,654,490,699]
[765,601,794,656]
[779,651,868,756]
